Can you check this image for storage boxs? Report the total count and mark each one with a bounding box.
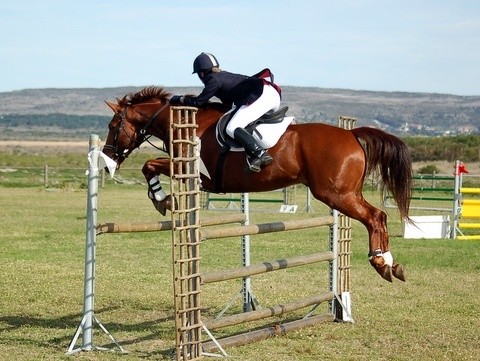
[402,215,451,239]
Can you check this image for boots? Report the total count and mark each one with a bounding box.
[234,127,272,172]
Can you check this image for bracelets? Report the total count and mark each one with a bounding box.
[180,97,185,104]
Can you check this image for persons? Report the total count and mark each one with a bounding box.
[169,52,281,175]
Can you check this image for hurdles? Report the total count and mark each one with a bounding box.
[381,160,480,240]
[64,106,357,361]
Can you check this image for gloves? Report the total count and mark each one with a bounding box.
[169,94,182,105]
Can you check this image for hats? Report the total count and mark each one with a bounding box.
[191,52,219,74]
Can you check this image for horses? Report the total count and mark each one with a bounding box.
[102,84,422,283]
